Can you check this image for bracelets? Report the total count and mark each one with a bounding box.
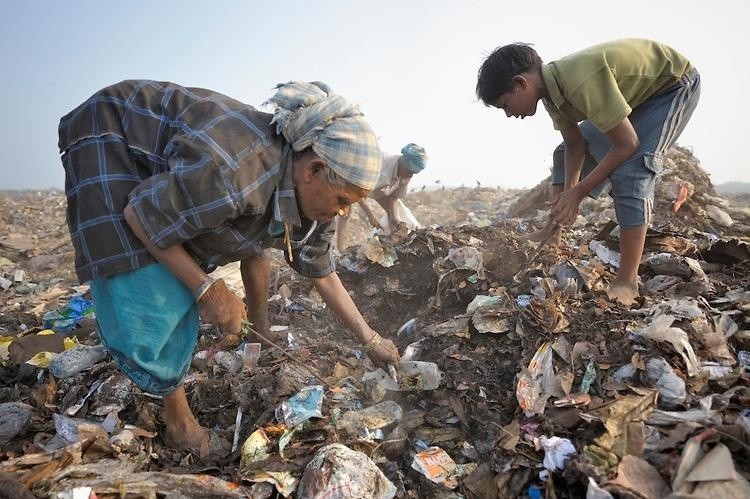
[192,277,216,304]
[362,333,383,354]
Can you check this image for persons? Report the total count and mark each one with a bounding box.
[335,143,429,251]
[472,36,702,311]
[55,76,402,461]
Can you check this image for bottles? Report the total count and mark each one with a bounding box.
[48,345,109,379]
[336,401,403,435]
[375,361,445,391]
